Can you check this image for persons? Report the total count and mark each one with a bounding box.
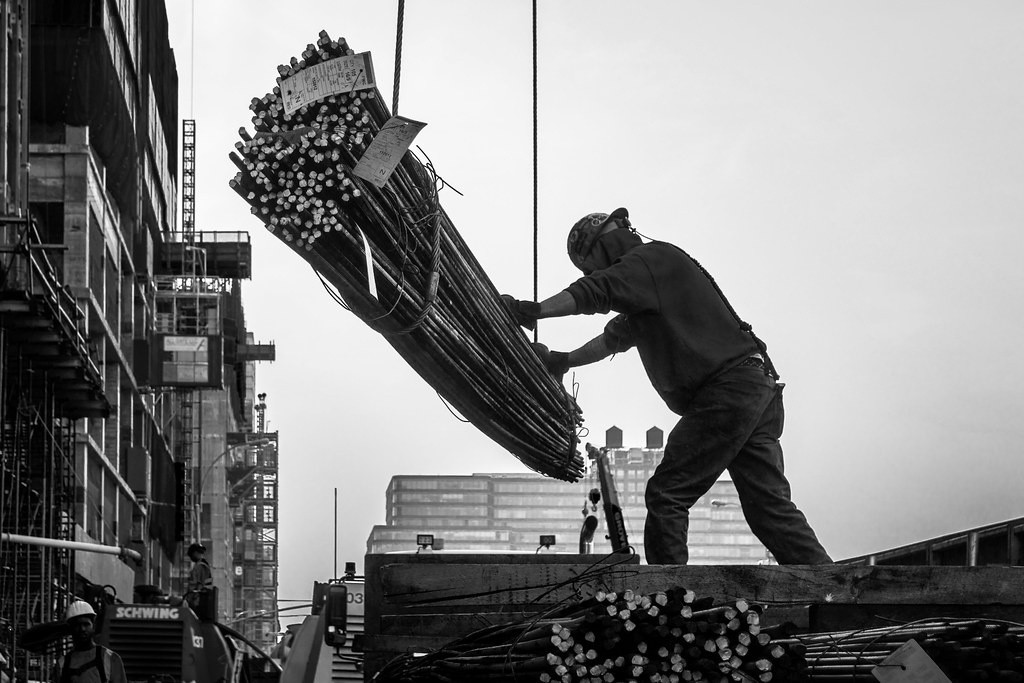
[499,208,834,564]
[184,543,214,610]
[48,601,128,683]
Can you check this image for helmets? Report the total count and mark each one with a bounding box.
[187,543,207,555]
[67,601,97,621]
[567,207,629,271]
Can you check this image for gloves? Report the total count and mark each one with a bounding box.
[531,342,570,375]
[500,294,541,331]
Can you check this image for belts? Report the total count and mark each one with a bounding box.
[737,357,764,368]
